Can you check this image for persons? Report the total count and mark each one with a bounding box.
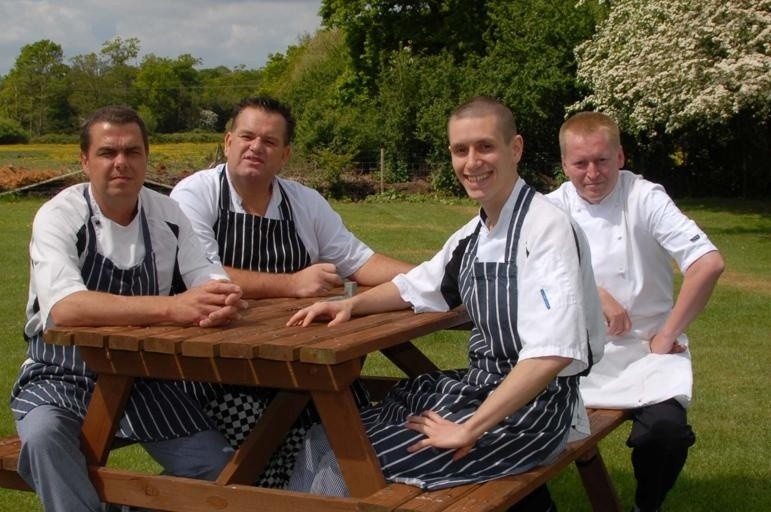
[160,92,427,482]
[542,107,729,508]
[6,105,239,512]
[275,93,610,496]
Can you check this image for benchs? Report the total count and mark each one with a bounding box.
[354,407,635,512]
[1,434,139,503]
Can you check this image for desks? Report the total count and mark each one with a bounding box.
[44,285,474,512]
